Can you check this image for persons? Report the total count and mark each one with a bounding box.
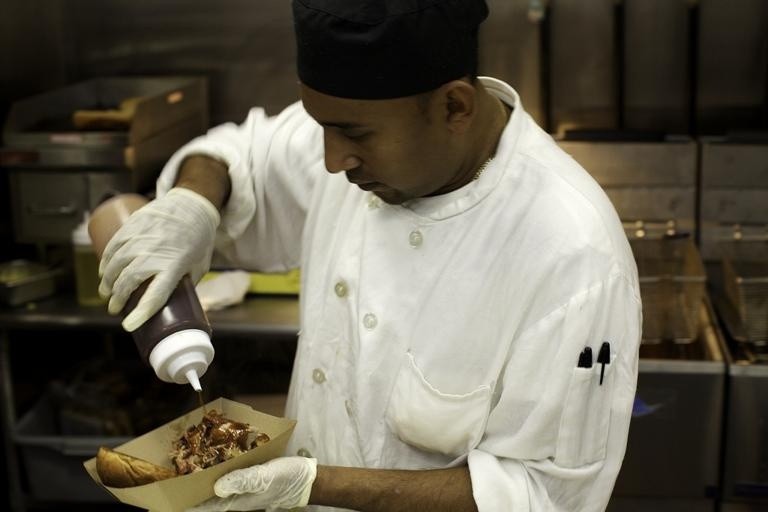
[98,0,643,512]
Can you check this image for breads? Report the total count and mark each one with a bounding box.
[95,446,175,487]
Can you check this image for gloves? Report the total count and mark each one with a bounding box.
[146,455,320,512]
[96,187,221,332]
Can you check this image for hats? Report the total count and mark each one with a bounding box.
[290,0,489,100]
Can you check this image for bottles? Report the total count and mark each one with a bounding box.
[85,193,215,393]
[70,208,108,309]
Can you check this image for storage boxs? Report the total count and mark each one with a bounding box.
[0,73,212,245]
